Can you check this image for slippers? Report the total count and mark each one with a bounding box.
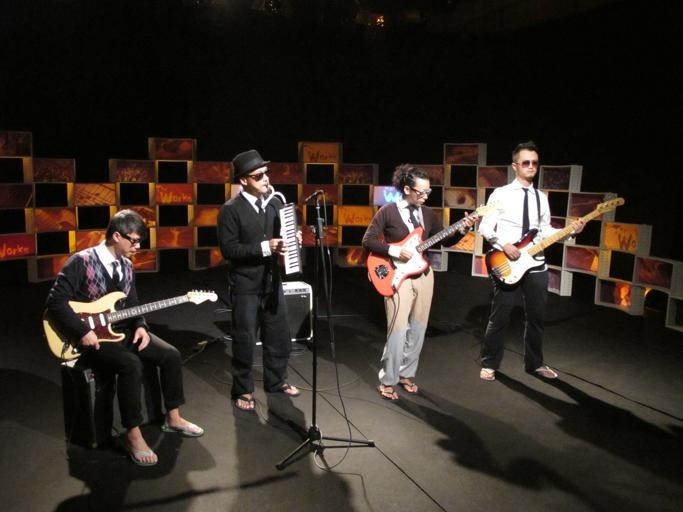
[160,419,204,438]
[125,441,159,467]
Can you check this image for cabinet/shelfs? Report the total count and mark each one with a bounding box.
[0,131,225,283]
[472,163,683,337]
[225,141,487,276]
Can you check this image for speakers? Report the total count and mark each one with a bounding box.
[284,282,316,342]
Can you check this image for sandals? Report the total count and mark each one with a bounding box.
[479,367,497,382]
[376,384,399,403]
[535,366,559,380]
[279,382,300,397]
[233,393,256,411]
[400,379,418,395]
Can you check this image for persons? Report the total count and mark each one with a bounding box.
[476,140,584,382]
[216,148,305,416]
[41,207,206,466]
[361,162,483,404]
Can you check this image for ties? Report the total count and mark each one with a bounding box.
[255,199,266,234]
[112,261,120,285]
[230,149,272,181]
[521,187,530,237]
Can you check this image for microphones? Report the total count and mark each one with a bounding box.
[302,190,321,205]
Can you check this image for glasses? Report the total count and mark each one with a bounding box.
[514,160,539,168]
[405,206,420,229]
[244,169,272,181]
[120,232,143,245]
[408,186,432,197]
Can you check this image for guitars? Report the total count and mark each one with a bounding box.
[43,289,218,361]
[367,201,490,298]
[485,196,624,285]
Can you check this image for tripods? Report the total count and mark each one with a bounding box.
[274,247,373,470]
